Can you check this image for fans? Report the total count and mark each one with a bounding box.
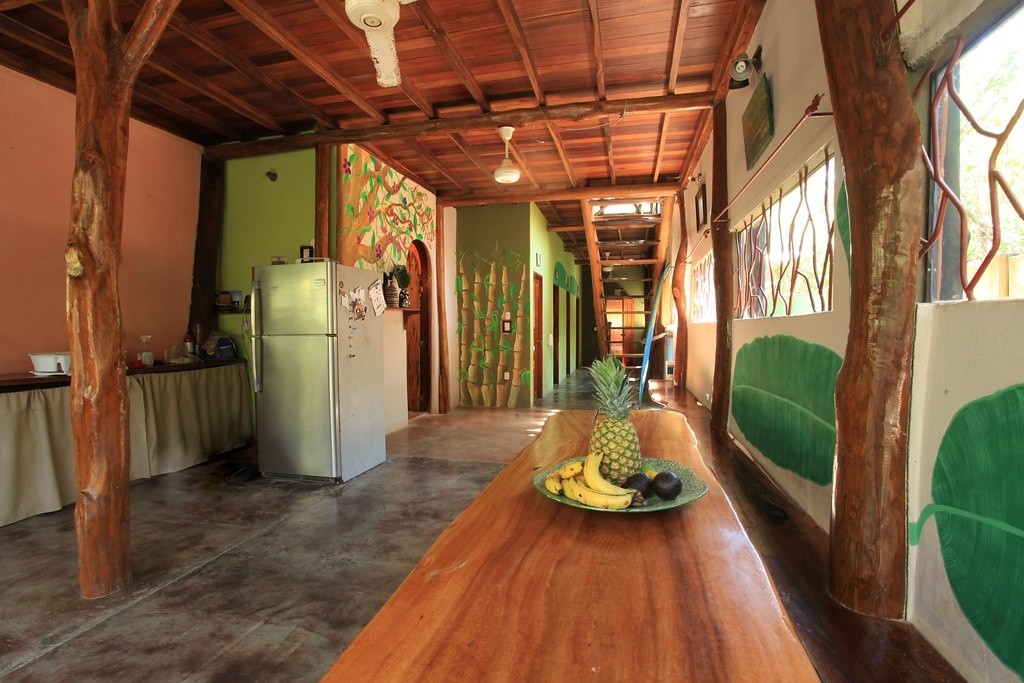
[344,1,419,87]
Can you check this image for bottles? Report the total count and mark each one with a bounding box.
[386,281,398,307]
[184,332,194,353]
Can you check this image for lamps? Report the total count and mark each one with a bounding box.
[494,126,520,184]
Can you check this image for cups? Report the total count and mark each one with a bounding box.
[400,289,410,308]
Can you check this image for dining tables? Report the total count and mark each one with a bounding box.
[316,409,825,683]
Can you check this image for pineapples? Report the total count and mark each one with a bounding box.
[582,355,643,486]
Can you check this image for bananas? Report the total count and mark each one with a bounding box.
[544,450,642,508]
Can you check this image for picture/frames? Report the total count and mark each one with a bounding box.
[695,183,707,234]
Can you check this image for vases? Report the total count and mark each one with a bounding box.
[398,289,411,309]
[386,281,399,308]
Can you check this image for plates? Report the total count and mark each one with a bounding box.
[533,456,708,512]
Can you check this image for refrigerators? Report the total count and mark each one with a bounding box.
[250,262,386,483]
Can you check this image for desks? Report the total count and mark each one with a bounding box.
[0,357,255,531]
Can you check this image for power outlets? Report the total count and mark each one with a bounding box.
[504,372,510,380]
[505,312,510,319]
[505,322,510,331]
